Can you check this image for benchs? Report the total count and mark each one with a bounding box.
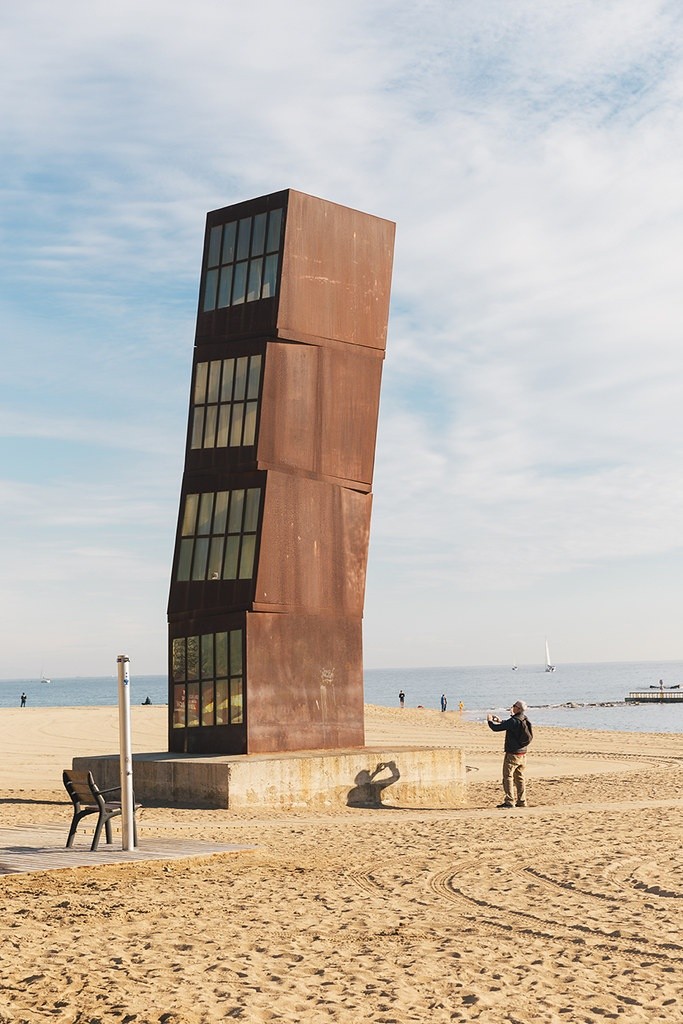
[62,768,141,851]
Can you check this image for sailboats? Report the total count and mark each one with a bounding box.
[544,635,557,673]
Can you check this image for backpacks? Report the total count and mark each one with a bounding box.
[513,718,533,747]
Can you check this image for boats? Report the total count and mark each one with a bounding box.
[40,672,51,684]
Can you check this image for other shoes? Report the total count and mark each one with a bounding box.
[515,803,526,807]
[496,802,513,808]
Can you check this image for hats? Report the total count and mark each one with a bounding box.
[514,701,526,711]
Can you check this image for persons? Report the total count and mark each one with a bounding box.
[487,700,533,808]
[398,690,406,708]
[20,692,27,707]
[458,701,464,714]
[142,696,152,705]
[440,694,447,712]
